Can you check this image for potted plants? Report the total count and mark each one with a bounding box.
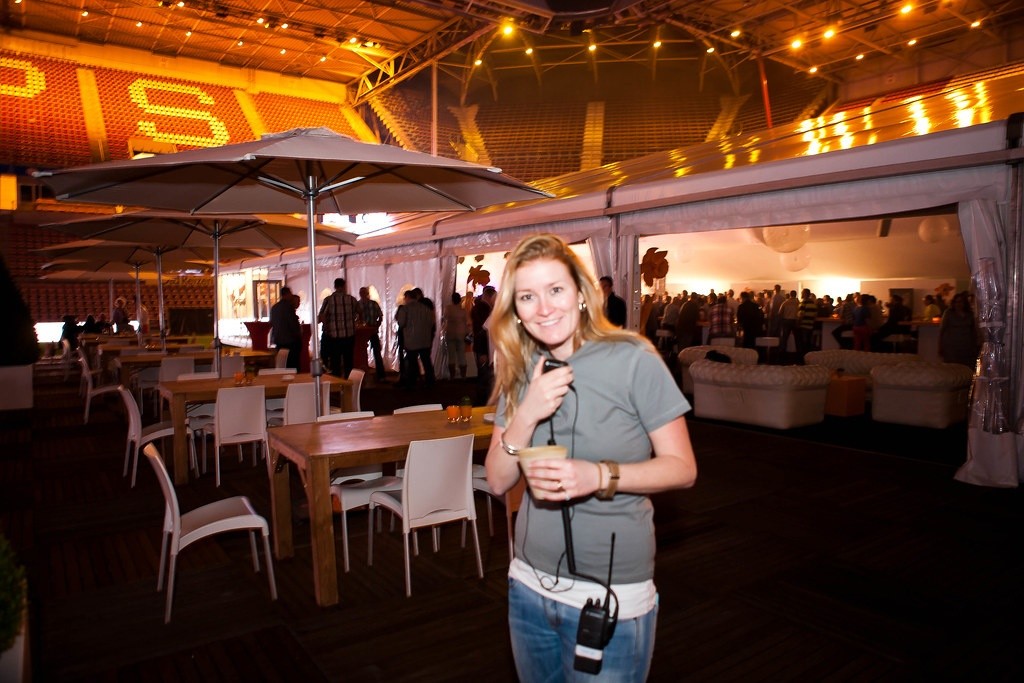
[0,532,33,683]
[0,251,42,410]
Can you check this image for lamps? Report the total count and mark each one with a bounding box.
[877,220,891,237]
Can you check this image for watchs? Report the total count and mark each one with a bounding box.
[594,459,619,500]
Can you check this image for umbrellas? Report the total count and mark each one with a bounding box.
[31,206,358,379]
[30,127,554,417]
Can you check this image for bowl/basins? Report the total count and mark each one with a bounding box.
[832,314,838,319]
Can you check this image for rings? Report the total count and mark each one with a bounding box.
[564,492,570,501]
[558,481,564,493]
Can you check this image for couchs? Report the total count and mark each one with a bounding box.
[679,345,758,395]
[689,360,829,429]
[872,361,973,428]
[803,351,922,389]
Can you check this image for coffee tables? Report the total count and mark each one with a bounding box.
[826,376,865,416]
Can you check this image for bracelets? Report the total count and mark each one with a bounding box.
[500,432,533,455]
[597,464,603,493]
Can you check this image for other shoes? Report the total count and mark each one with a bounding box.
[375,372,387,380]
[395,376,438,387]
[445,378,471,384]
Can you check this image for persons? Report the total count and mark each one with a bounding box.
[485,233,696,683]
[111,298,135,335]
[84,315,97,335]
[58,314,83,352]
[96,313,112,335]
[270,277,497,389]
[641,284,978,383]
[598,276,627,330]
[132,300,149,335]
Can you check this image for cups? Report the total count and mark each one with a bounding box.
[224,347,230,356]
[447,405,461,423]
[245,373,254,384]
[517,445,568,499]
[836,367,844,377]
[461,405,472,422]
[234,372,243,384]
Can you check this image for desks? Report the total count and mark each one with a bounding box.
[112,350,271,421]
[912,320,944,361]
[266,405,497,607]
[77,332,161,341]
[96,343,203,393]
[157,373,353,487]
[80,336,188,381]
[815,316,842,351]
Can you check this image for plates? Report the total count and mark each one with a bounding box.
[483,413,496,422]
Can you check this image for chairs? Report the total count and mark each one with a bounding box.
[65,331,524,624]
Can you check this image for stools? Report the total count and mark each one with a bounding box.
[710,338,735,346]
[656,330,673,358]
[841,331,854,338]
[885,334,907,352]
[756,337,779,364]
